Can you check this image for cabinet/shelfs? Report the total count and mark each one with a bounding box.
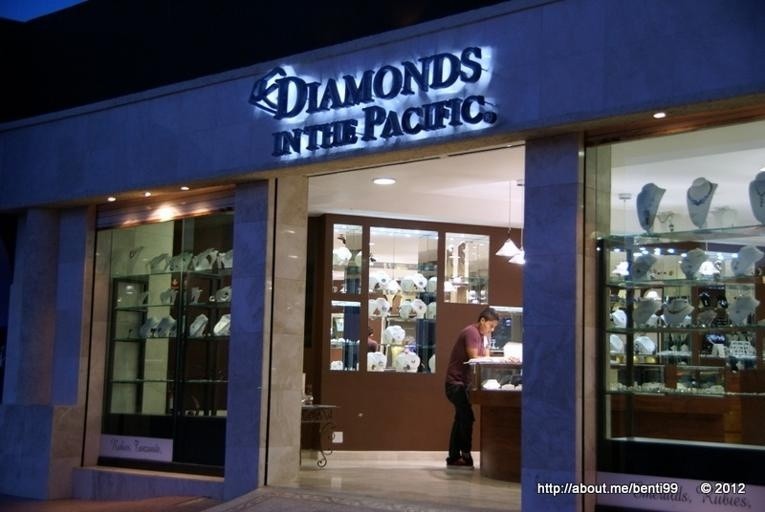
[464,355,523,486]
[298,404,341,470]
[98,267,232,476]
[593,224,765,489]
[304,213,522,452]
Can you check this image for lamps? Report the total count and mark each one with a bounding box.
[495,148,521,257]
[508,180,525,264]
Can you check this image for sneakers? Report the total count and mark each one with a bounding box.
[446,452,474,470]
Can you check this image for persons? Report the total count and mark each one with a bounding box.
[368,326,378,351]
[444,307,499,468]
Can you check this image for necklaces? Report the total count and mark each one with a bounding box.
[754,180,765,208]
[686,259,702,267]
[734,301,752,313]
[397,335,401,338]
[381,278,386,283]
[382,304,387,311]
[633,266,644,275]
[667,306,688,315]
[734,254,743,270]
[412,358,416,363]
[418,279,424,286]
[375,355,385,366]
[640,192,660,225]
[688,181,712,205]
[157,324,164,332]
[418,304,424,310]
